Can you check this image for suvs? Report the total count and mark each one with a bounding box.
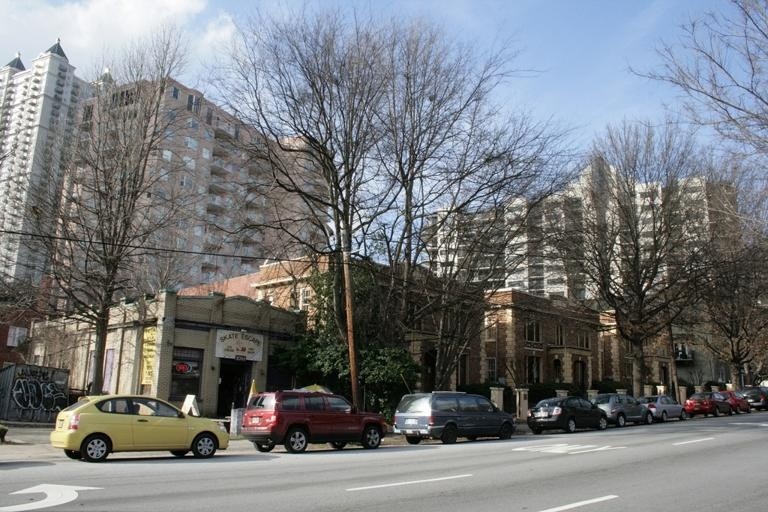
[740,386,768,411]
[240,391,390,454]
[394,390,517,445]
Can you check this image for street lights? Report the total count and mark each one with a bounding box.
[123,290,158,396]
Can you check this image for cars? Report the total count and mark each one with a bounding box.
[685,391,733,417]
[49,393,230,463]
[636,395,688,421]
[589,392,654,427]
[718,390,751,416]
[526,396,607,431]
